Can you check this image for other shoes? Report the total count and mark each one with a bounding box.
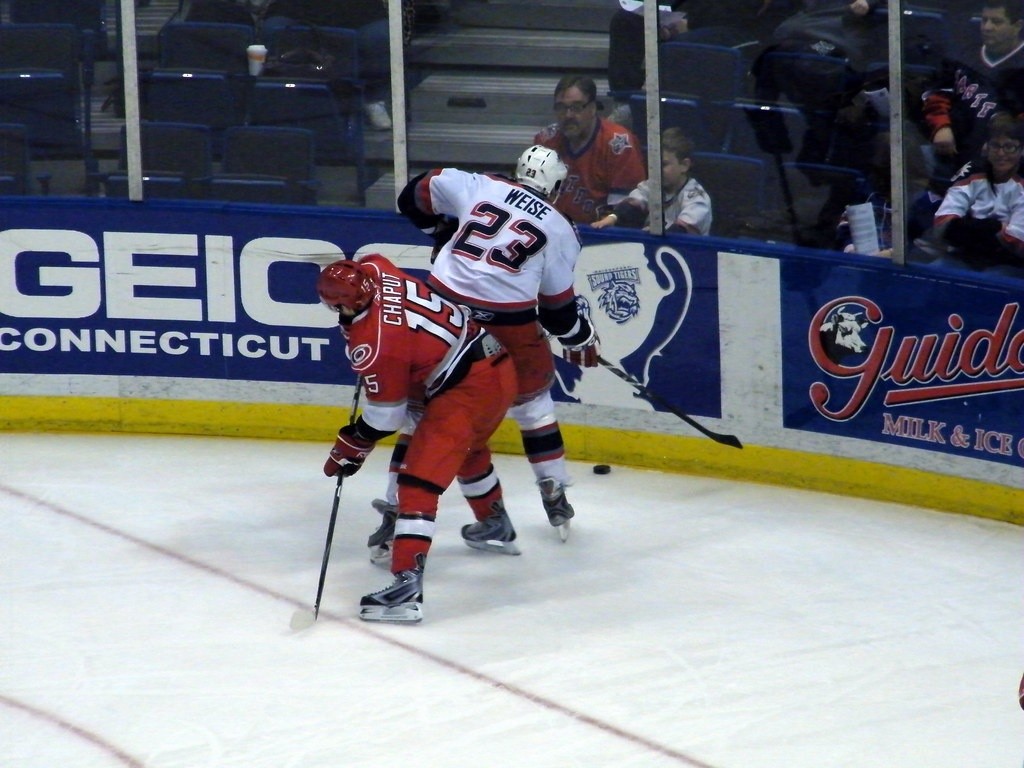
[609,101,632,131]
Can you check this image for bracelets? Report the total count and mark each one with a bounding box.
[609,213,617,219]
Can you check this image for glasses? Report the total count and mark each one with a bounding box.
[554,100,592,113]
[986,141,1024,153]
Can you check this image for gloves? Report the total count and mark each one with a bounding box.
[324,424,376,477]
[555,314,601,368]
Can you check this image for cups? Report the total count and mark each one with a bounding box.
[246,44,267,76]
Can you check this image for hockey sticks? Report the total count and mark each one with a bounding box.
[769,145,807,234]
[312,377,364,623]
[599,356,743,449]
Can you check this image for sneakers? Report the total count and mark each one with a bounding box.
[536,476,574,541]
[363,99,391,130]
[367,498,399,563]
[462,502,521,555]
[358,552,427,622]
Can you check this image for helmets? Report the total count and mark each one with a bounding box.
[317,260,373,310]
[514,145,569,204]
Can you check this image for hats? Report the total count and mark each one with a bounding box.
[556,74,605,110]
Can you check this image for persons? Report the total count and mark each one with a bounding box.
[225,0,414,131]
[609,0,1024,277]
[312,253,519,623]
[368,144,600,565]
[590,124,712,235]
[533,73,647,227]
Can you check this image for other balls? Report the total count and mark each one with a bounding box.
[594,464,611,474]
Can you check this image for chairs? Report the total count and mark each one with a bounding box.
[608,3,984,251]
[0,20,365,198]
